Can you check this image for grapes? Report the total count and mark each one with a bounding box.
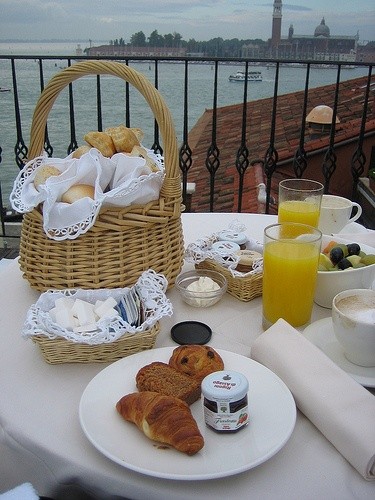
[328,244,375,269]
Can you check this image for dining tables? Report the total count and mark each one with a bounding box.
[0,212,375,500]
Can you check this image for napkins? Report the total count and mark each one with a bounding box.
[251,318,375,482]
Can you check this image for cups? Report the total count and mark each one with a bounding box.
[332,288,375,368]
[304,194,362,236]
[278,179,324,240]
[261,222,324,333]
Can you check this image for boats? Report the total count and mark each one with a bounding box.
[228,71,263,82]
[0,87,12,92]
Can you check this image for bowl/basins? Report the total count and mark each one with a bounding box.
[175,269,228,308]
[296,233,375,309]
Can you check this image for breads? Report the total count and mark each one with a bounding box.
[33,165,61,191]
[168,345,224,382]
[61,183,94,203]
[136,362,202,406]
[115,392,204,456]
[72,125,160,173]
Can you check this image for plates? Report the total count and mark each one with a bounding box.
[339,221,369,234]
[303,317,375,388]
[79,345,297,481]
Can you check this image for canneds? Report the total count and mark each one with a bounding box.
[201,370,249,432]
[211,232,262,274]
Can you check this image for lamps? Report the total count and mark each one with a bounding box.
[305,105,340,134]
